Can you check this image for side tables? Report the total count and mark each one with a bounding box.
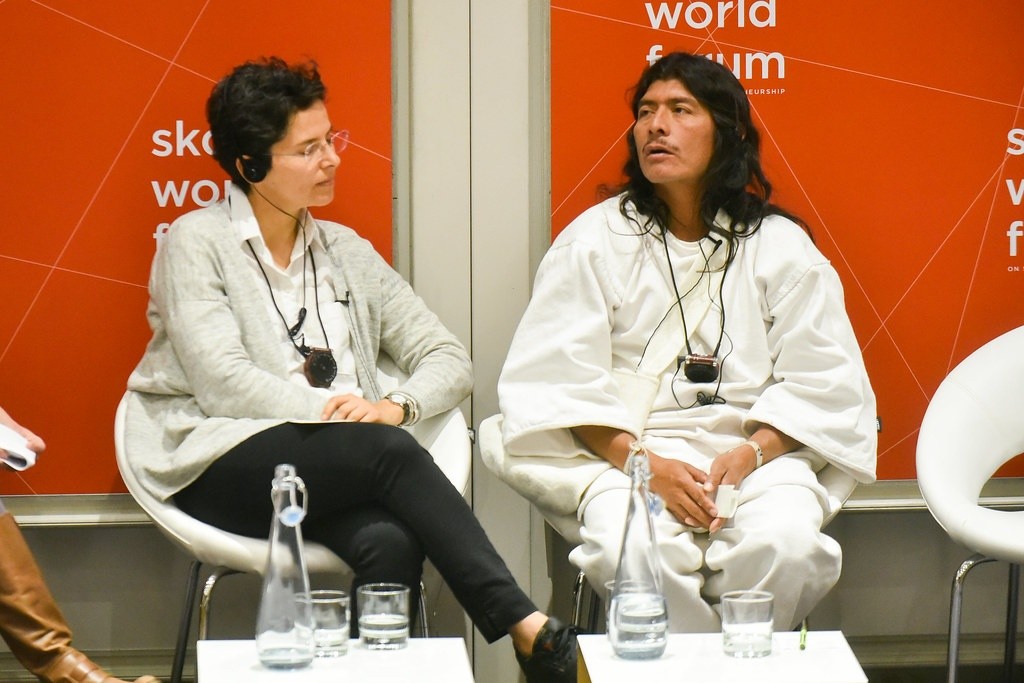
[194,636,476,683]
[576,627,871,683]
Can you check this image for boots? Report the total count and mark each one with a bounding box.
[0,512,163,683]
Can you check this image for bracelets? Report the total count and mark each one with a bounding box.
[742,440,763,469]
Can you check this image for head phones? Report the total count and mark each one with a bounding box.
[732,95,749,145]
[223,65,267,184]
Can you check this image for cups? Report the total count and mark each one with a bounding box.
[719,589,774,659]
[358,584,409,652]
[294,590,350,658]
[601,579,650,640]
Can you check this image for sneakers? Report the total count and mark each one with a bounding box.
[514,618,593,683]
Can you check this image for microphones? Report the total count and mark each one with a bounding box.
[334,290,349,305]
[703,233,723,255]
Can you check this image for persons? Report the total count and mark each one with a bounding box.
[0,405,160,683]
[496,51,880,632]
[119,52,591,683]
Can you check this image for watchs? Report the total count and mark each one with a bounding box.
[384,394,412,428]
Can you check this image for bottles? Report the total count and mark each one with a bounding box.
[607,455,671,660]
[252,464,316,672]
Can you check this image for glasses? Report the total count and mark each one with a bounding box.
[259,130,349,160]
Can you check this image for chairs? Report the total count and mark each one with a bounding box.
[112,348,474,683]
[914,323,1023,682]
